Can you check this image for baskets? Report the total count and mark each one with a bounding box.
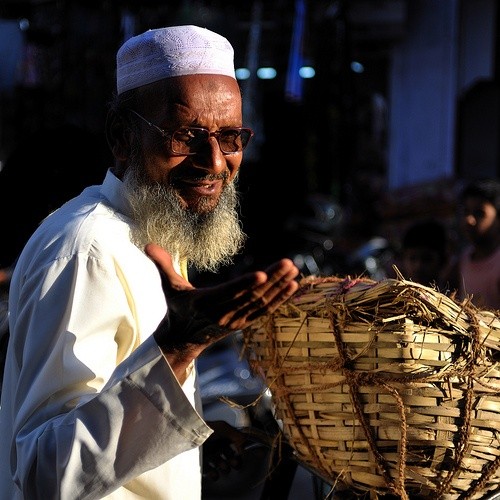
[243,271,500,500]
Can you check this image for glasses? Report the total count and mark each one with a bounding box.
[120,106,253,157]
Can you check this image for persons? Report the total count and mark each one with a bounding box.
[454,183,500,311]
[1,23,300,499]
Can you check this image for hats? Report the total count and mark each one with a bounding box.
[117,24,236,96]
